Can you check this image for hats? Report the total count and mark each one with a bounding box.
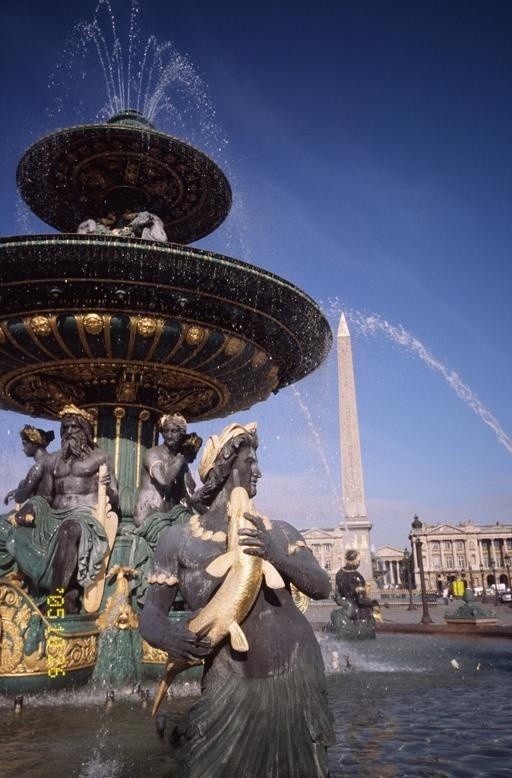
[473,582,511,600]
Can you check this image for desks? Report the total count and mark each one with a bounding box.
[490,557,500,607]
[410,510,435,626]
[503,551,512,608]
[439,560,488,603]
[402,547,417,611]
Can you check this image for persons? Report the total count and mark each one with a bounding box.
[122,209,167,242]
[133,413,195,544]
[335,551,381,621]
[7,405,121,598]
[1,425,54,562]
[140,425,332,778]
